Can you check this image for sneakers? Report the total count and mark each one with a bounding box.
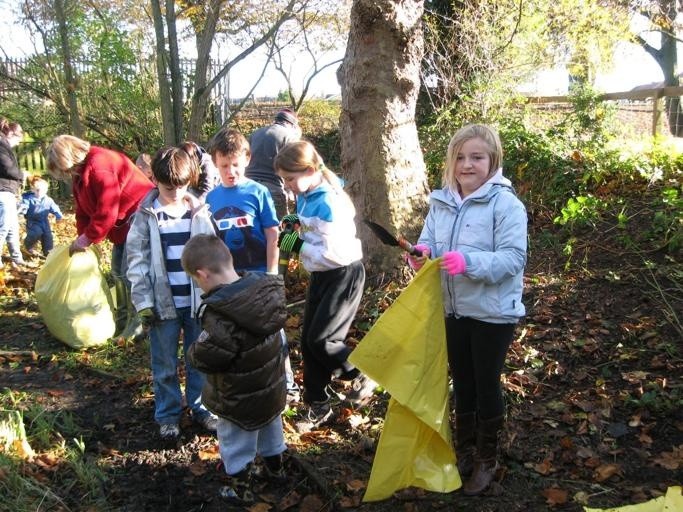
[159,424,179,439]
[293,403,333,433]
[197,414,218,432]
[343,372,377,410]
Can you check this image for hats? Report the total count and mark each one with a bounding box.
[274,108,298,124]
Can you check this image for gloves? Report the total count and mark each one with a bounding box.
[281,214,300,226]
[138,308,155,327]
[405,245,430,270]
[277,230,304,254]
[442,251,465,275]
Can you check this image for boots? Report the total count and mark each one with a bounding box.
[251,453,287,486]
[455,412,475,480]
[114,280,145,346]
[217,464,254,506]
[464,416,501,495]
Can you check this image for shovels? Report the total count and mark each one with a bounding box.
[363,219,422,257]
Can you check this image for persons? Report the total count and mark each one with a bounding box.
[246,108,302,277]
[272,140,373,431]
[45,133,159,349]
[176,140,223,199]
[134,152,156,186]
[406,123,529,498]
[0,116,32,268]
[21,175,62,259]
[125,147,219,440]
[181,231,289,506]
[204,127,303,405]
